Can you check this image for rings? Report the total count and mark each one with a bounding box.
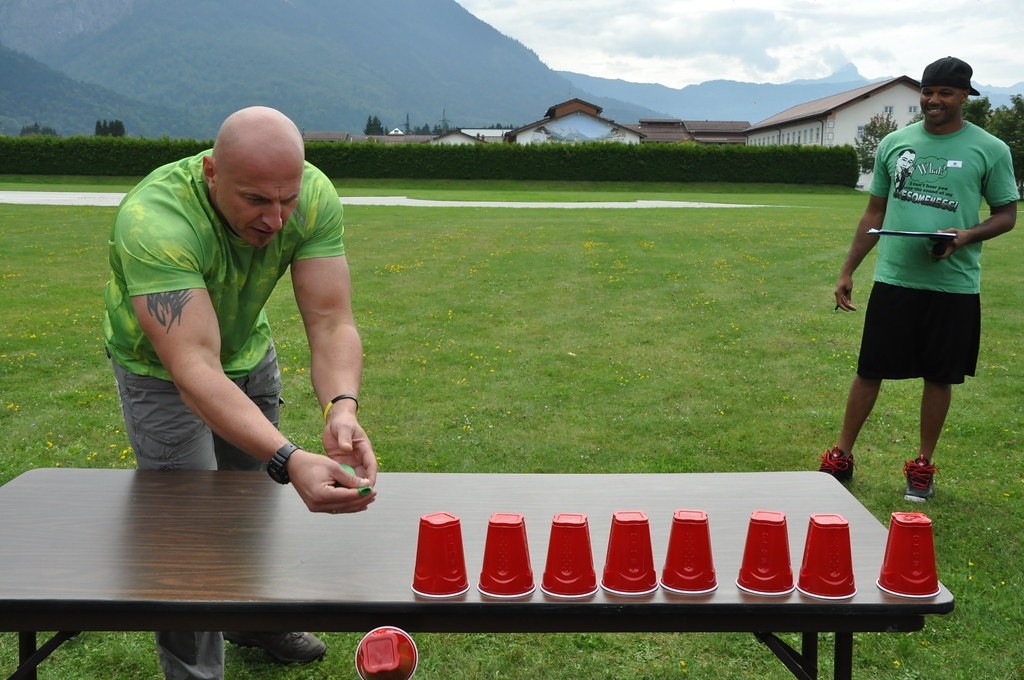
[333,510,336,514]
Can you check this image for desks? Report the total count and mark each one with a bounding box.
[0,467,955,680]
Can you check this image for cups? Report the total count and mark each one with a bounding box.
[876,512,942,599]
[540,514,599,599]
[476,513,537,600]
[735,511,796,596]
[659,510,718,595]
[599,511,660,596]
[410,513,470,601]
[355,626,418,680]
[795,516,857,599]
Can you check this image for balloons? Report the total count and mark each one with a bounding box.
[340,464,372,496]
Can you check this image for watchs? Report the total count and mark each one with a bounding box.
[267,441,302,485]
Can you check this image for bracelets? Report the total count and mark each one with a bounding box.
[323,393,359,426]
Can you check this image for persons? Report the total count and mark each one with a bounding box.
[103,106,376,680]
[817,56,1021,503]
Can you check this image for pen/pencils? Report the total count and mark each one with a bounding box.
[833,288,851,313]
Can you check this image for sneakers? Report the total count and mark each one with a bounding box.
[819,445,853,481]
[903,453,939,503]
[223,629,324,664]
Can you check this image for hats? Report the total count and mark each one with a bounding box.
[921,55,981,97]
